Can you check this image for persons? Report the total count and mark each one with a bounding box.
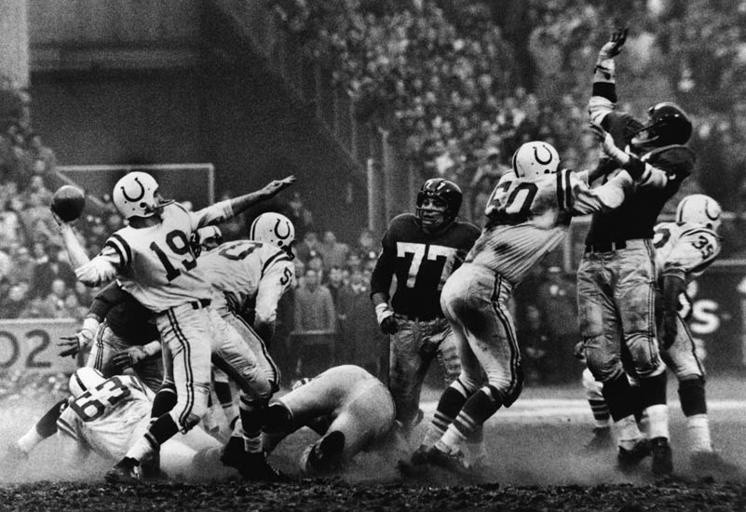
[258,0,397,168]
[406,139,641,485]
[365,177,498,479]
[0,78,58,169]
[576,19,698,478]
[568,194,737,478]
[397,2,746,384]
[0,169,397,485]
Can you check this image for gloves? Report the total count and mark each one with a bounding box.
[110,339,163,372]
[372,300,401,337]
[594,25,630,62]
[56,318,100,359]
[658,303,684,351]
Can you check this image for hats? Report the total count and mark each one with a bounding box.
[44,241,62,253]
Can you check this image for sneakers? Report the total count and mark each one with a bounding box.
[614,435,676,478]
[0,442,32,482]
[574,425,615,460]
[687,449,739,479]
[228,402,290,433]
[102,464,151,491]
[410,441,501,493]
[313,430,346,477]
[236,449,293,488]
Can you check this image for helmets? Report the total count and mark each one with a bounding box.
[415,176,464,225]
[673,190,726,234]
[643,101,693,147]
[111,169,161,221]
[196,223,227,251]
[510,139,564,180]
[248,209,298,257]
[67,366,107,399]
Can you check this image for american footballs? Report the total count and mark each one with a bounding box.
[51,185,85,223]
